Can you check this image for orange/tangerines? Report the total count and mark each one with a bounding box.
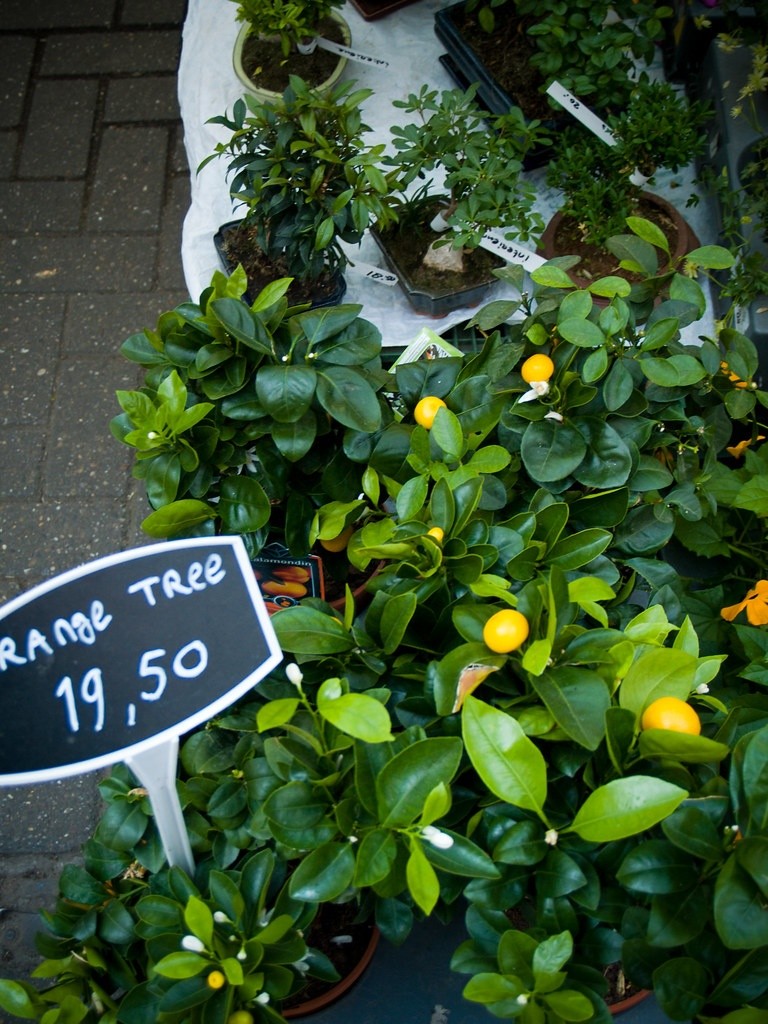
[642,696,701,737]
[320,522,353,551]
[414,396,446,429]
[426,527,443,540]
[521,353,554,384]
[483,609,528,653]
[227,1009,255,1024]
[207,970,224,989]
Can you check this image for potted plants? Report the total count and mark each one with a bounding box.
[368,81,555,321]
[223,0,355,103]
[430,0,673,172]
[199,75,406,321]
[538,77,718,308]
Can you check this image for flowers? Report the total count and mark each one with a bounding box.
[0,214,768,1024]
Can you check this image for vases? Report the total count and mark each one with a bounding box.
[263,876,384,1018]
[491,851,658,1015]
[262,491,395,616]
